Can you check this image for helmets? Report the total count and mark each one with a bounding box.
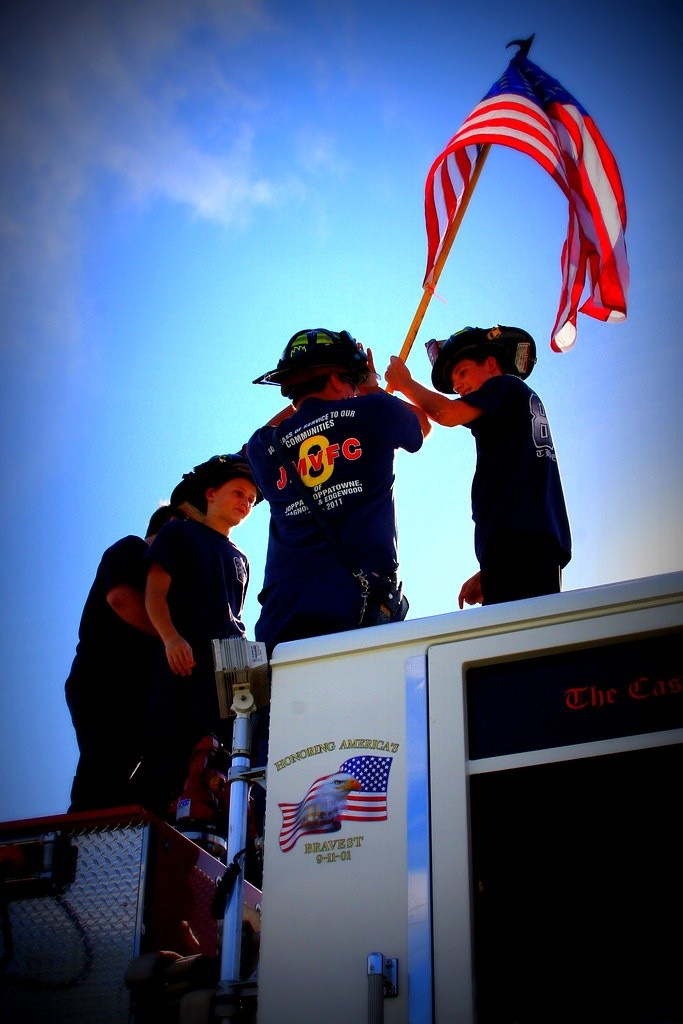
[252,329,370,398]
[424,325,537,394]
[170,449,265,515]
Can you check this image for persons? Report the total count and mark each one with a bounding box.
[143,453,266,839]
[388,325,573,609]
[246,328,432,888]
[63,501,184,813]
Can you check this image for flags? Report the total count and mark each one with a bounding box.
[422,51,631,353]
